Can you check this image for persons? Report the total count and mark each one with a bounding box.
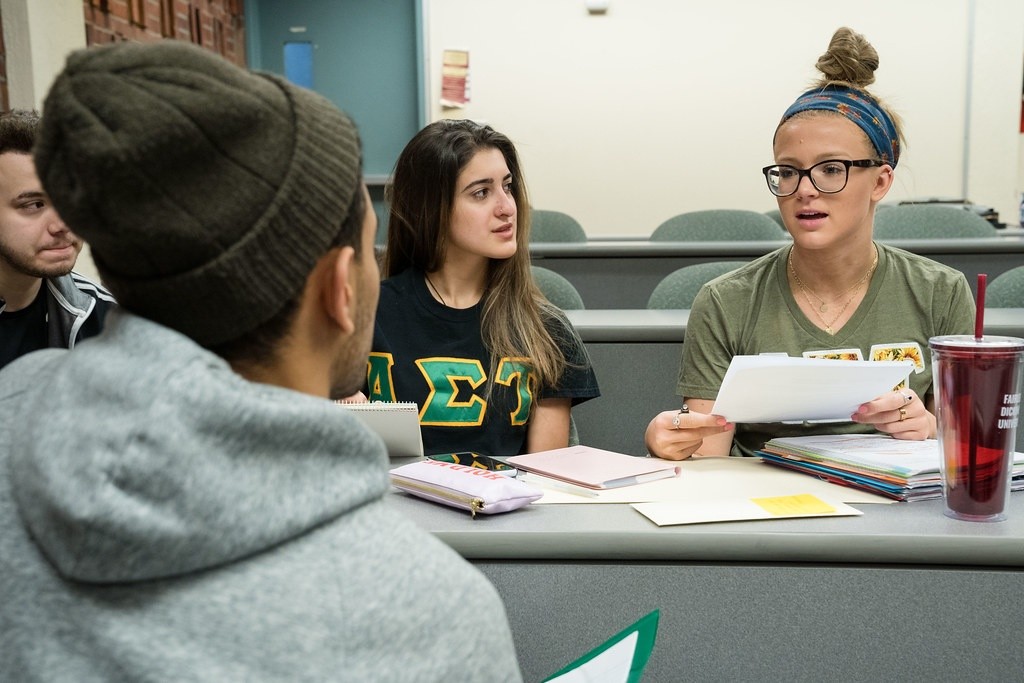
[335,118,601,452]
[644,27,976,462]
[0,110,119,370]
[0,40,521,683]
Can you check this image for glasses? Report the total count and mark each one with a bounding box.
[763,159,892,197]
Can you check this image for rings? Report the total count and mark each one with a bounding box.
[900,407,907,421]
[899,389,913,407]
[672,412,681,430]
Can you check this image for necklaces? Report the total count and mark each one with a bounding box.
[426,275,447,306]
[789,244,879,336]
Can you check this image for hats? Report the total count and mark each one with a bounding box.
[31,40,362,345]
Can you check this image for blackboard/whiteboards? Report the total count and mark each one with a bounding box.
[414,1,1024,257]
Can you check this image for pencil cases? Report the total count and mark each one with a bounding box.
[389,460,544,520]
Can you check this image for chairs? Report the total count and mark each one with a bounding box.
[529,265,584,310]
[875,204,998,235]
[646,260,748,310]
[985,266,1024,309]
[529,208,586,240]
[656,209,785,240]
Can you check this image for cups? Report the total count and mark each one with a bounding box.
[927,334,1024,524]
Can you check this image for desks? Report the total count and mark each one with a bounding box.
[528,237,1024,310]
[565,310,1023,457]
[392,455,1024,683]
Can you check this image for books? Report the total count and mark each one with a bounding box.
[754,435,1024,503]
[332,401,424,457]
[426,452,518,478]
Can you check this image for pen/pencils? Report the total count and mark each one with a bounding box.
[682,407,694,456]
